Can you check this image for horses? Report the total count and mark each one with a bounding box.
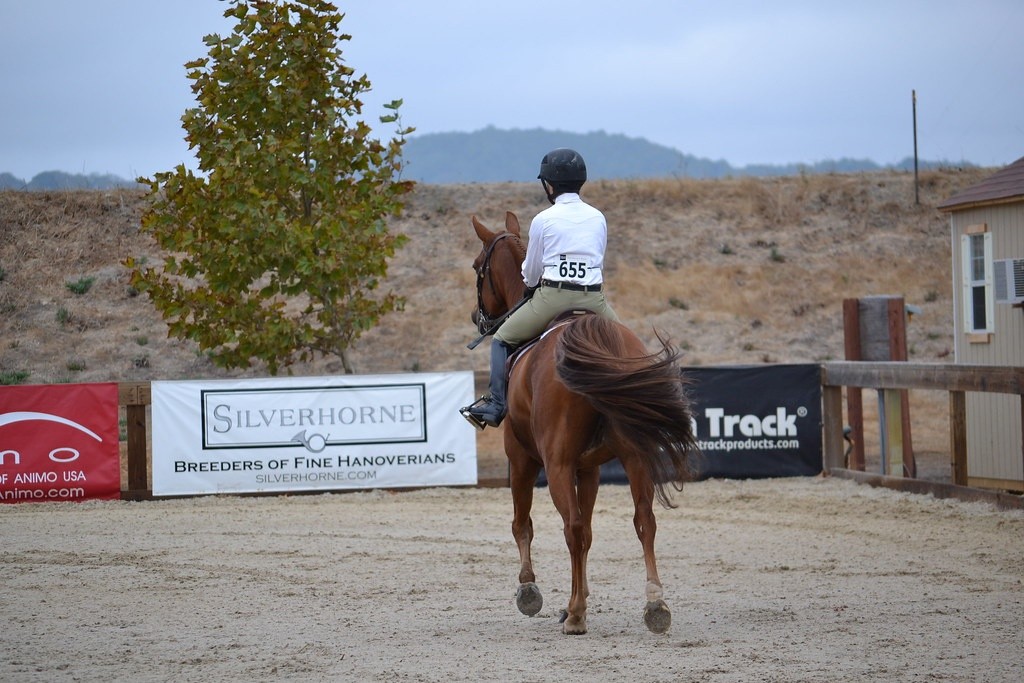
[470,211,706,635]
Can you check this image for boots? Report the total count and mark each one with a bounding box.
[459,339,523,428]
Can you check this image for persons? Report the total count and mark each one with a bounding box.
[459,148,623,428]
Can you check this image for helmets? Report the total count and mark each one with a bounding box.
[538,147,588,194]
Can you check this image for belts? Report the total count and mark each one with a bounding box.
[541,279,602,293]
